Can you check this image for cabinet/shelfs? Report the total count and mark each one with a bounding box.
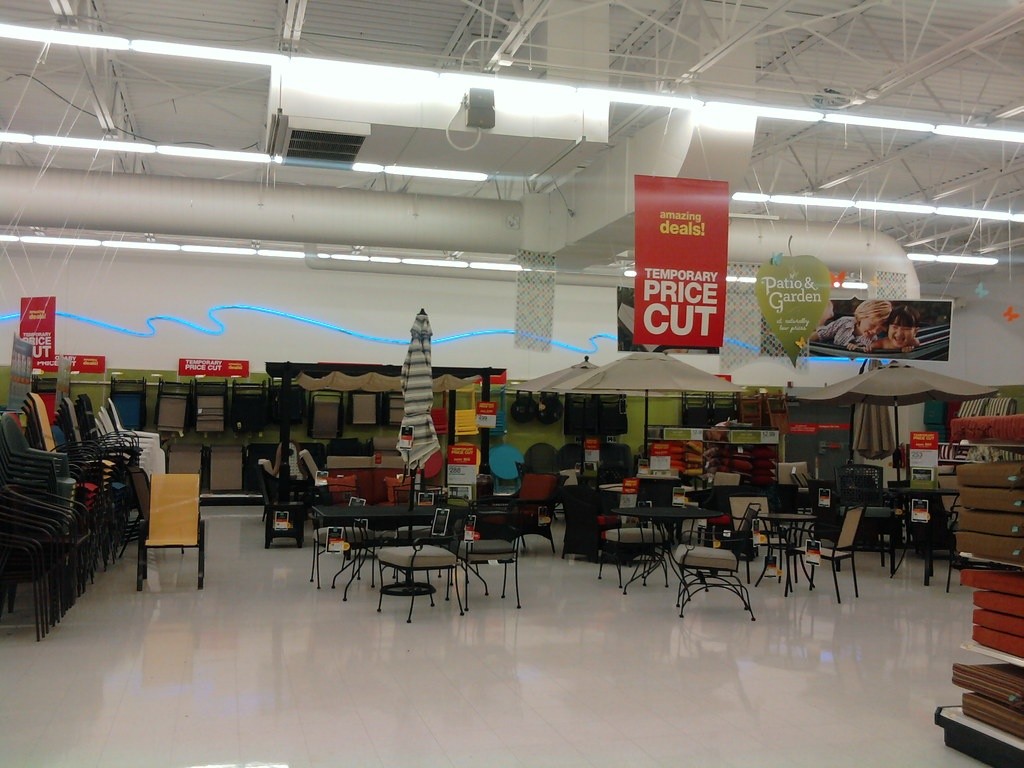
[935,442,1024,768]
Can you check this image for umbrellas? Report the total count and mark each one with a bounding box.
[852,359,896,472]
[553,352,748,474]
[504,354,664,489]
[399,308,441,583]
[796,360,1001,480]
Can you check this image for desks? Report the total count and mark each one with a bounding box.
[886,487,960,577]
[310,505,470,589]
[610,506,723,596]
[753,512,817,596]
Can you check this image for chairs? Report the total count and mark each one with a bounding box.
[0,378,1024,643]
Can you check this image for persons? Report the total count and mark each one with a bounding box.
[811,300,921,353]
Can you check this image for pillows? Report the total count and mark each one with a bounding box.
[748,458,776,469]
[707,465,751,477]
[705,457,753,473]
[752,476,778,484]
[750,446,778,458]
[702,446,753,460]
[750,467,775,477]
